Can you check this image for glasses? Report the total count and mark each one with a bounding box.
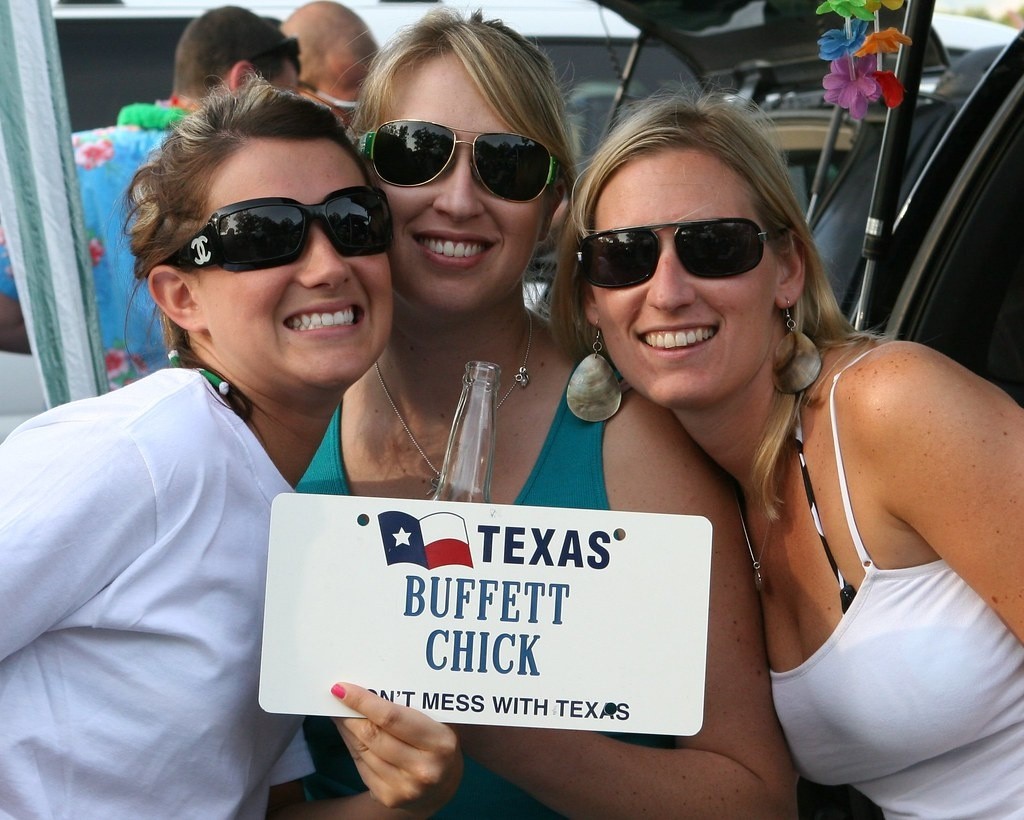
[576,217,786,288]
[245,39,300,63]
[355,119,556,204]
[166,186,394,272]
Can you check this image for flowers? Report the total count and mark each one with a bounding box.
[817,1,909,118]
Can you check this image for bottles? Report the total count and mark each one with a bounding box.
[431,361,503,505]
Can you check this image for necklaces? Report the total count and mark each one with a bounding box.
[737,497,772,589]
[374,308,532,495]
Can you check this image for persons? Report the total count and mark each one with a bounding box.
[554,102,1023,820]
[0,83,461,819]
[274,9,798,820]
[0,5,303,391]
[278,0,377,130]
